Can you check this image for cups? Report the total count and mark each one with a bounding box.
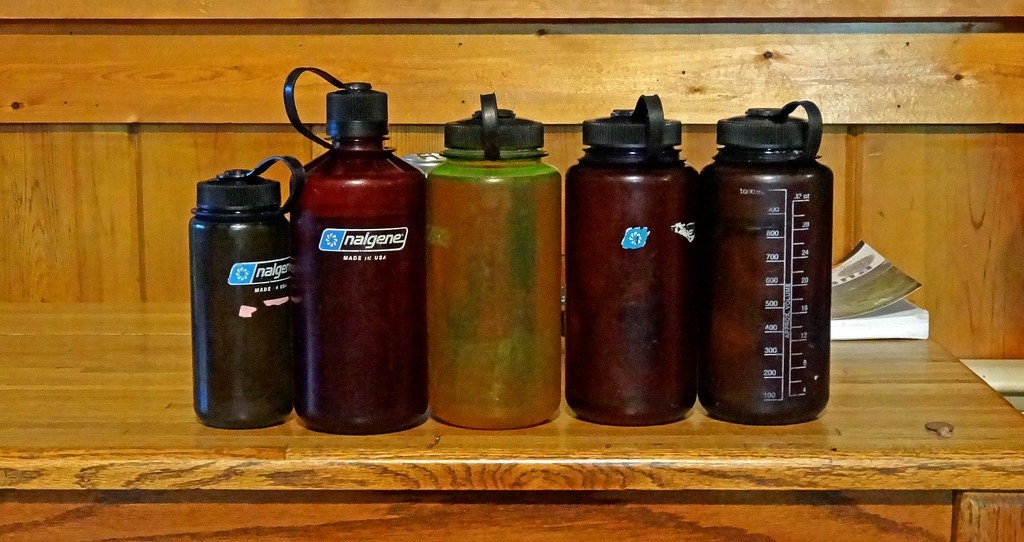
[427,93,561,430]
[188,155,305,430]
[565,94,701,427]
[698,100,834,426]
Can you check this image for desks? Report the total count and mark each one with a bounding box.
[0,302,1024,542]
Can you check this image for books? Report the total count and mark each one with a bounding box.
[831,240,929,340]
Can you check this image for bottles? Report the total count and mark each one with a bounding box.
[283,66,428,434]
